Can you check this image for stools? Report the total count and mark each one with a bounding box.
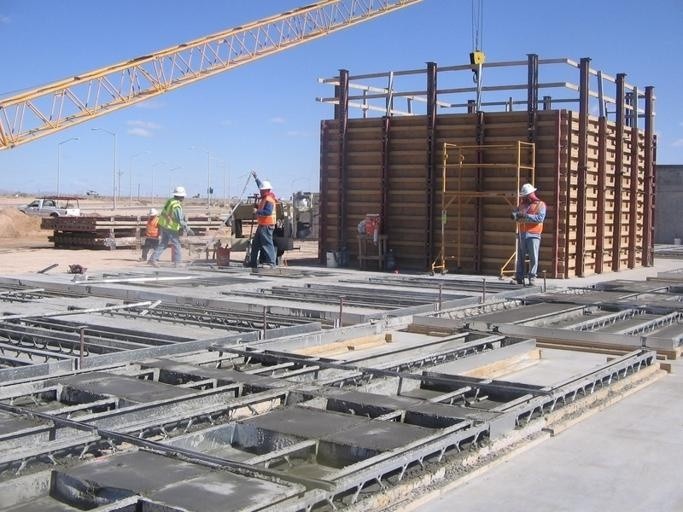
[356,234,388,272]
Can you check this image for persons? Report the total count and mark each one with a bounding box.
[138,208,159,262]
[511,183,546,286]
[148,187,190,268]
[251,169,276,269]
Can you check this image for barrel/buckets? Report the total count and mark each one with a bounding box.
[674,238,681,245]
[366,214,380,234]
[385,249,396,270]
[326,251,340,267]
[217,247,230,265]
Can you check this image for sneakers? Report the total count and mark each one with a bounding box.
[529,276,536,286]
[510,278,525,285]
[147,259,160,268]
[137,257,147,262]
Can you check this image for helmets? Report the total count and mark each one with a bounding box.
[519,184,537,195]
[147,208,158,216]
[257,181,273,190]
[171,187,187,196]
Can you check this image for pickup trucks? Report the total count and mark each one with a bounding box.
[17,199,80,218]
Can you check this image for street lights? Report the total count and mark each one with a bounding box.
[55,135,80,198]
[91,126,119,210]
[125,144,236,204]
